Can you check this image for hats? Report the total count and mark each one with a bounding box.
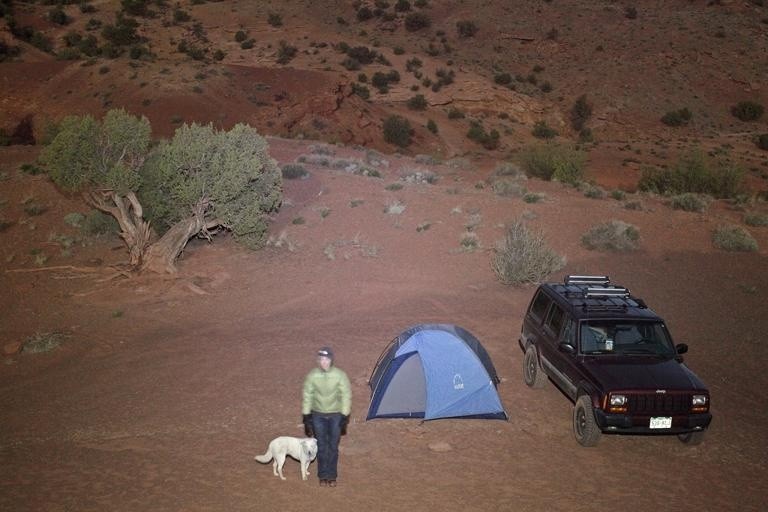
[318,347,334,361]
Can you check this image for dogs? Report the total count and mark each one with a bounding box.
[254,436,318,480]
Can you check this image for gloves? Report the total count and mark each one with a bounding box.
[303,414,315,437]
[339,414,351,436]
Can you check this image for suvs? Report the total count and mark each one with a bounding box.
[518,275,712,447]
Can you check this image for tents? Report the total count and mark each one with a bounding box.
[366,324,509,423]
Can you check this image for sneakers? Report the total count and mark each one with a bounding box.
[320,479,336,487]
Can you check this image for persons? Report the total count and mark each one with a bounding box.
[302,348,353,487]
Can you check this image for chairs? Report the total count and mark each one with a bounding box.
[581,322,653,348]
